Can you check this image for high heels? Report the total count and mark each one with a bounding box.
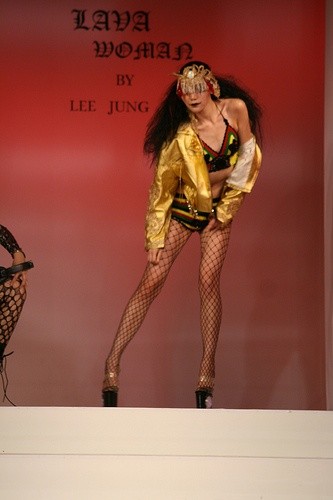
[195,376,214,406]
[102,373,118,406]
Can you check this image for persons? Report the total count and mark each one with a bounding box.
[0,222,34,408]
[97,58,262,409]
